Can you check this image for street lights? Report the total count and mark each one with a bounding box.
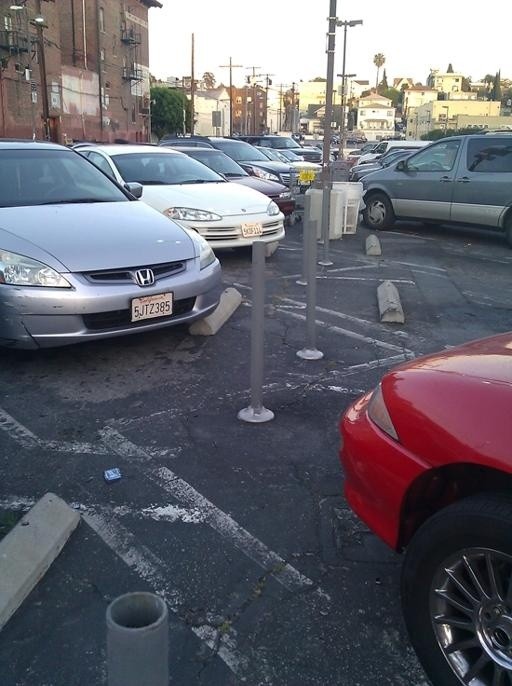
[334,19,364,158]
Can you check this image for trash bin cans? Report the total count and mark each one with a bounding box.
[306,181,363,243]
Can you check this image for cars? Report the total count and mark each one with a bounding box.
[335,325,512,686]
[344,131,512,242]
